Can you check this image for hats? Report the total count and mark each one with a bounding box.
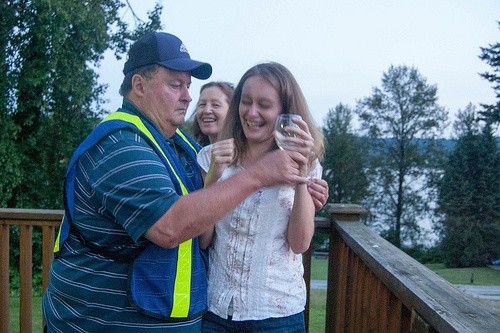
[122,32,213,80]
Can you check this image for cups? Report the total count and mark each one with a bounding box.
[274,113,303,151]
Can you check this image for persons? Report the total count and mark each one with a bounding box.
[42,32,329,333]
[194,61,326,332]
[182,81,236,147]
